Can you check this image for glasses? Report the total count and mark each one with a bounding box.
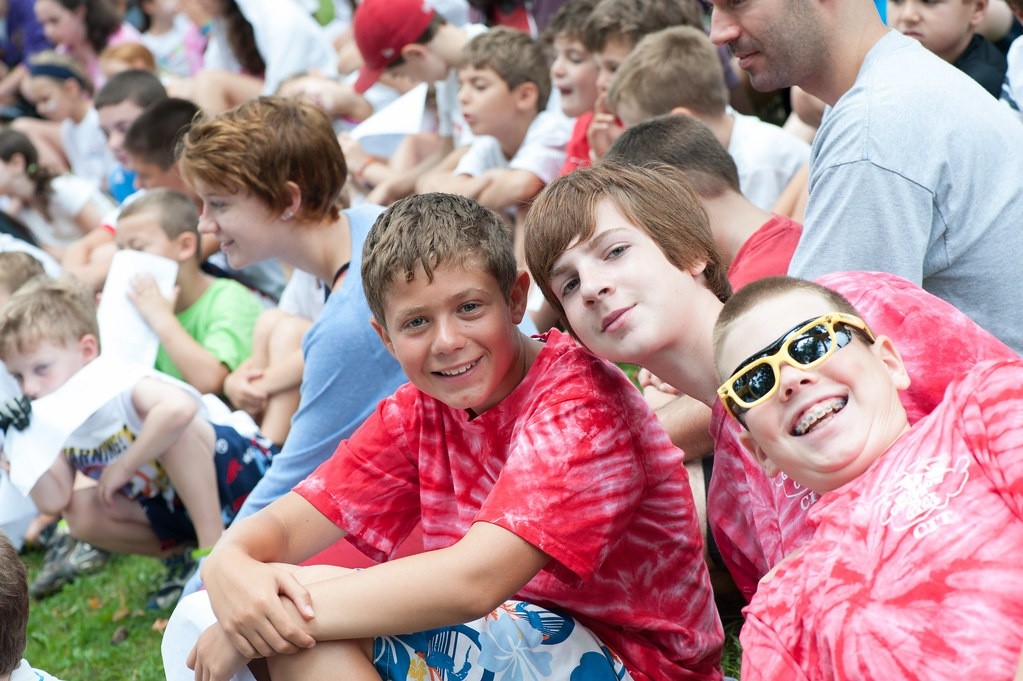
[717,312,874,423]
[725,316,872,430]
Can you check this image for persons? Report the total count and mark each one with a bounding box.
[0,0,1023,681]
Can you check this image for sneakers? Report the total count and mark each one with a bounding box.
[67,540,110,572]
[148,558,199,613]
[31,534,76,597]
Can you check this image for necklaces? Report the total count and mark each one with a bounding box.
[330,262,349,291]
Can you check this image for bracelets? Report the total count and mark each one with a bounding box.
[353,156,382,183]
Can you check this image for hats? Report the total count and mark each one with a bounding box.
[355,0,437,93]
